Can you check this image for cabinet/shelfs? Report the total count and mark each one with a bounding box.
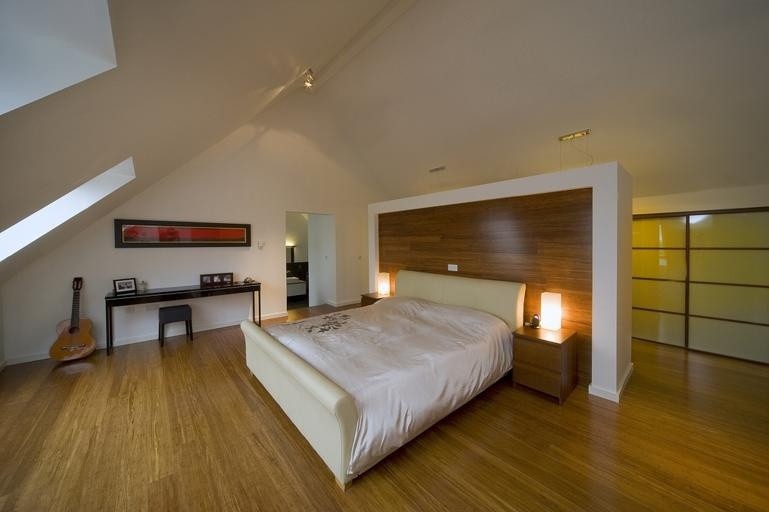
[286,274,307,297]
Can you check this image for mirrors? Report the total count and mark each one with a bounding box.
[286,246,295,264]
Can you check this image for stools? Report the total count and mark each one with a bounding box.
[153,304,193,347]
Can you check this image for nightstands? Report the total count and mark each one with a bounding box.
[358,291,388,307]
[512,325,581,405]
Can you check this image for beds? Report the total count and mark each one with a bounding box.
[239,270,529,494]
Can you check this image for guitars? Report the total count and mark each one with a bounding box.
[48,277,96,361]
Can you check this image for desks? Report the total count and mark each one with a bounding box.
[103,277,264,354]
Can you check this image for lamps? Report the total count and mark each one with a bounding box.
[536,291,564,332]
[303,67,314,88]
[376,271,392,295]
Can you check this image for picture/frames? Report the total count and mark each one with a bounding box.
[112,217,252,249]
[111,278,138,295]
